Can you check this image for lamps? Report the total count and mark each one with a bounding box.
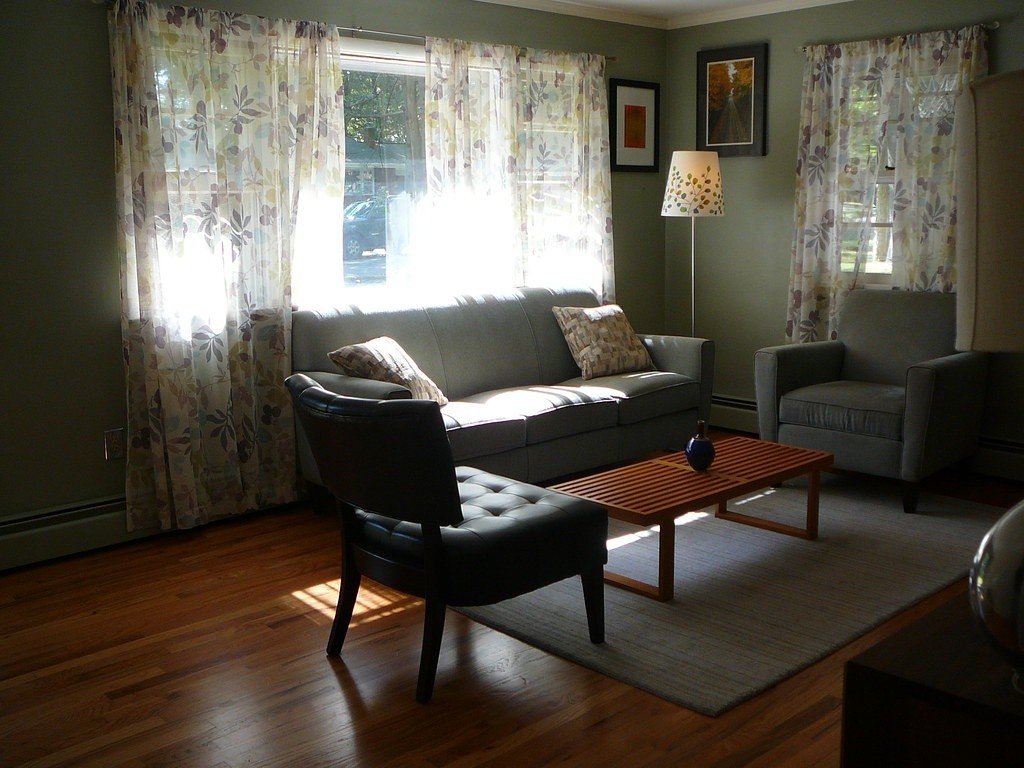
[661,150,726,338]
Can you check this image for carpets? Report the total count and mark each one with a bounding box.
[446,472,1011,719]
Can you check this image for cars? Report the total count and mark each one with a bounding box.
[344,197,385,261]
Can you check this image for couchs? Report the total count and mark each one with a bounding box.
[254,284,716,517]
[754,289,987,513]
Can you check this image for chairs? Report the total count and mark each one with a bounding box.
[280,371,610,708]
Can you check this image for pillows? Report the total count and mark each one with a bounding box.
[551,304,658,381]
[327,335,449,408]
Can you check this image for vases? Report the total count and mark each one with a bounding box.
[684,420,716,473]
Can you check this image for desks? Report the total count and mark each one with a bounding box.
[836,588,1024,768]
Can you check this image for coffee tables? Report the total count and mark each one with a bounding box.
[547,437,835,602]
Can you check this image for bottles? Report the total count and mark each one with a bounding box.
[685,420,715,472]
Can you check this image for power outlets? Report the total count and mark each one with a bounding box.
[104,427,125,461]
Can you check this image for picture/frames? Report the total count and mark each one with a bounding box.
[607,77,660,173]
[694,42,767,158]
